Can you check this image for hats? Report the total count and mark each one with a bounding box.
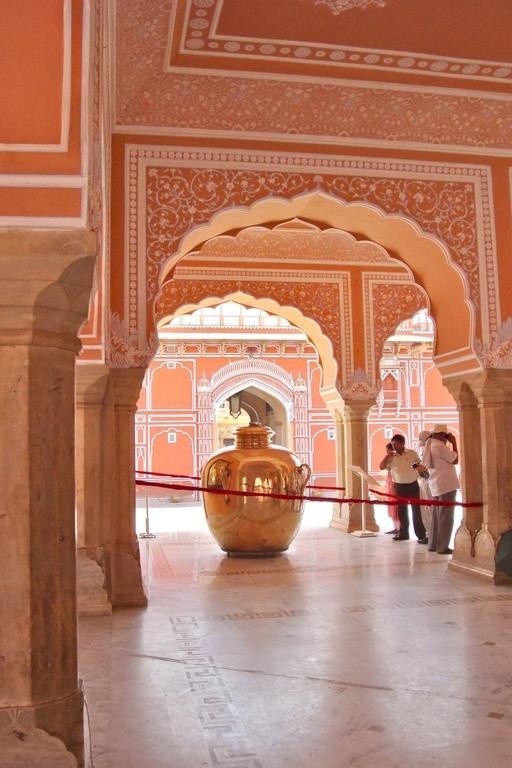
[429,424,448,434]
[419,430,432,446]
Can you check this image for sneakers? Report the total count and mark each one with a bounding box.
[387,529,409,540]
[418,536,426,542]
[428,548,453,554]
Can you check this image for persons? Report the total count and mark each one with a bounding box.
[384,442,402,534]
[426,422,461,554]
[416,429,432,544]
[379,434,425,544]
